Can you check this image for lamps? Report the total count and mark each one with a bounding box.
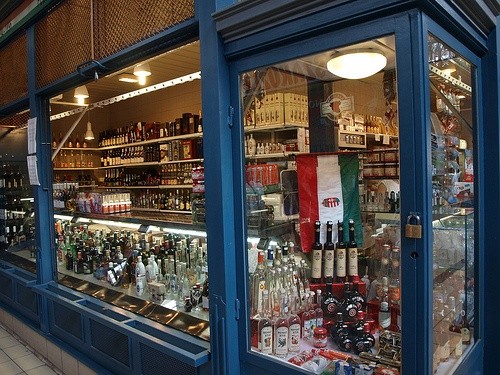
[118,62,152,85]
[73,85,89,99]
[326,47,387,80]
[84,83,95,140]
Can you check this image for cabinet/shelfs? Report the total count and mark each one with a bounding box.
[51,123,464,234]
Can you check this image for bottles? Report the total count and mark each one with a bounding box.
[239,38,476,375]
[0,167,35,258]
[52,109,210,317]
